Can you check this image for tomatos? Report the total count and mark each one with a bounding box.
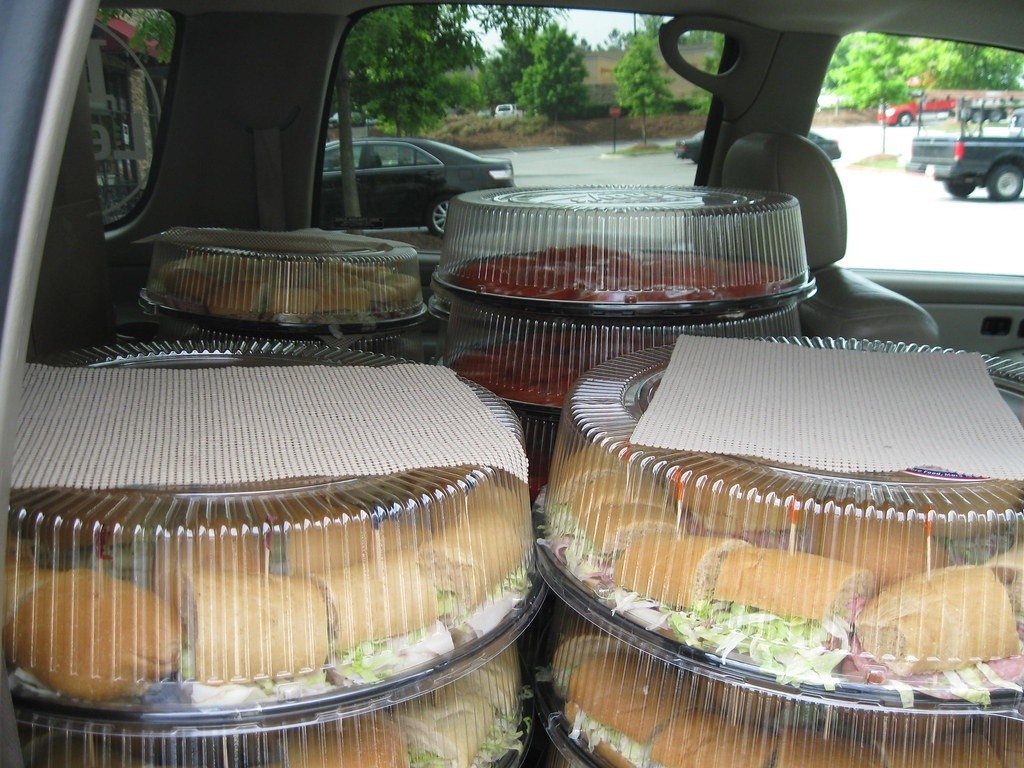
[443,247,780,407]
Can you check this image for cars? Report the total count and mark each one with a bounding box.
[877,93,957,128]
[316,137,517,238]
[495,104,523,121]
[674,130,842,165]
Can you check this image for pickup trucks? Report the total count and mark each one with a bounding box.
[906,106,1024,202]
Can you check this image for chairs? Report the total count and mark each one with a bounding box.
[723,132,939,347]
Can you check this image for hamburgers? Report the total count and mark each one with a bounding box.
[0,419,1024,768]
[149,258,420,325]
